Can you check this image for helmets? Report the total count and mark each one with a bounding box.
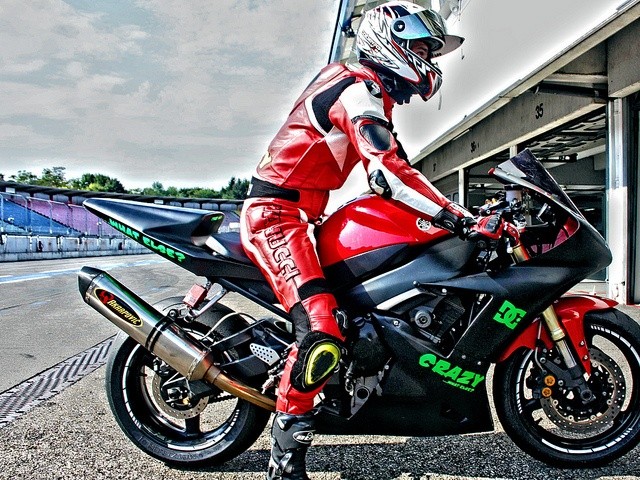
[346,0,465,105]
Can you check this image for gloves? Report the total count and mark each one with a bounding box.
[466,214,520,247]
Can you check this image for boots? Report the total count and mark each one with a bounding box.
[266,411,316,480]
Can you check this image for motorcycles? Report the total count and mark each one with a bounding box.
[77,148,640,470]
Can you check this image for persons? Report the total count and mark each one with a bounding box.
[240,0,519,480]
